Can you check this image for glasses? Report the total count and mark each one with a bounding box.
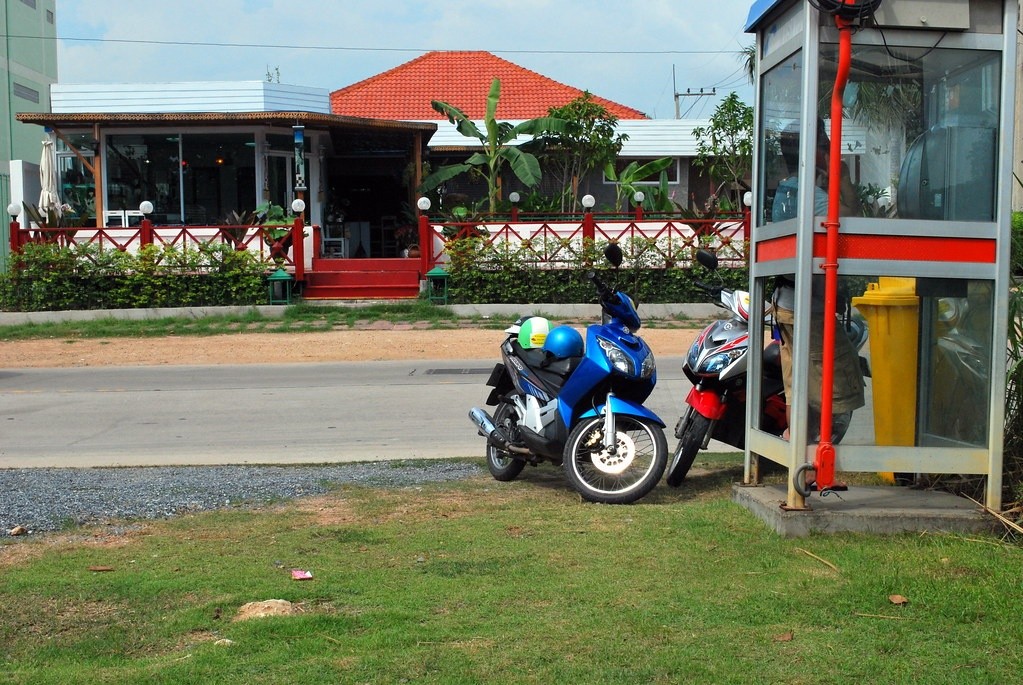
[816,138,831,153]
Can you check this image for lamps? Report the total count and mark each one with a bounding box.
[292,118,306,144]
[510,192,520,207]
[417,197,432,215]
[7,203,21,222]
[634,191,644,206]
[742,192,753,210]
[292,198,306,217]
[140,200,153,220]
[582,195,595,212]
[44,122,54,134]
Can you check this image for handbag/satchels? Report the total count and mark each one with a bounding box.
[776,276,848,315]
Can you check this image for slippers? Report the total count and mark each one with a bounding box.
[809,479,849,492]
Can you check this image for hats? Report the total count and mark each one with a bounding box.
[504,316,532,334]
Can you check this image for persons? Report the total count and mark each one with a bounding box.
[759,114,867,493]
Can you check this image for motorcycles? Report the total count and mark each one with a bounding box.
[466,244,669,502]
[665,249,874,488]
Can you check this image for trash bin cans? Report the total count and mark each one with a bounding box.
[852,273,922,486]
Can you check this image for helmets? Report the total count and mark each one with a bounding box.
[541,325,584,359]
[518,316,553,349]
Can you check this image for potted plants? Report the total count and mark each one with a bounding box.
[672,200,739,266]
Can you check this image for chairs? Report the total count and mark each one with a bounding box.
[319,221,345,258]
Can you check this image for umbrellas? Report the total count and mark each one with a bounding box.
[39,140,69,226]
